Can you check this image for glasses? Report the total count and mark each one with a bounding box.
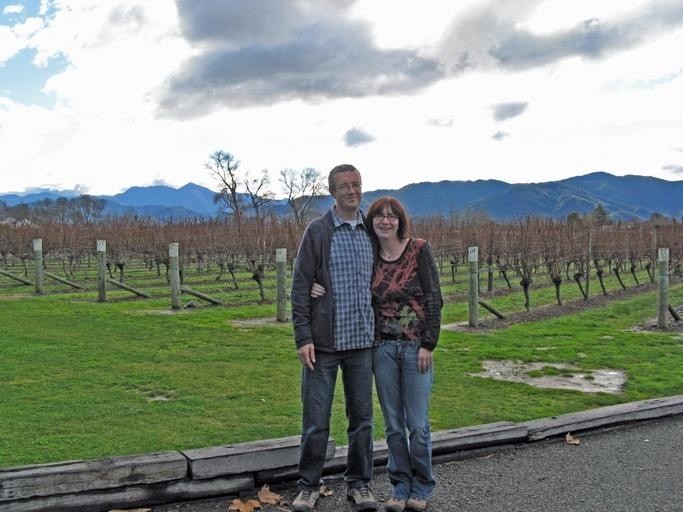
[372,213,400,222]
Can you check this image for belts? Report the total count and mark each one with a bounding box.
[374,330,407,341]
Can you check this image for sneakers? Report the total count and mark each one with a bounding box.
[406,493,427,511]
[291,478,324,512]
[345,477,379,511]
[384,495,406,512]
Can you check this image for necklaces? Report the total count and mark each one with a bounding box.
[384,252,398,261]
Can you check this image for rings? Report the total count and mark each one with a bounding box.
[422,362,426,367]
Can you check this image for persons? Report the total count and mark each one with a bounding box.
[309,195,444,511]
[288,163,385,511]
